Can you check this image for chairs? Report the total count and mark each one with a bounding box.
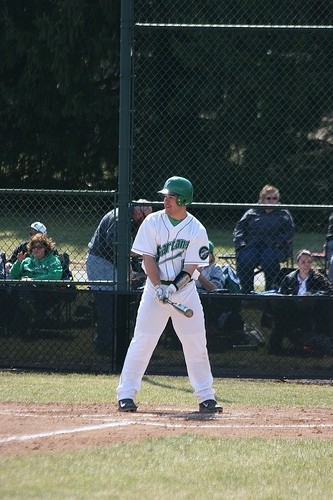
[233,235,294,274]
[0,250,333,348]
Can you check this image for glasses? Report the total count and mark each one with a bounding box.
[31,245,46,250]
[265,196,279,200]
[140,210,146,218]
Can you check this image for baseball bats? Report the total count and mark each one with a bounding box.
[164,298,194,318]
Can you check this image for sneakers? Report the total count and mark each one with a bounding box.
[199,399,223,412]
[117,398,138,412]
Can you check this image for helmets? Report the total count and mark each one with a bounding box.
[157,176,193,207]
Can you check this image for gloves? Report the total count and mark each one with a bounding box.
[155,286,164,302]
[164,284,176,304]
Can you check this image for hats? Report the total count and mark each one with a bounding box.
[208,240,214,255]
[30,221,46,233]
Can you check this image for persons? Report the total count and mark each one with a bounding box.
[86,198,152,354]
[5,221,58,274]
[116,176,223,413]
[270,249,333,355]
[9,232,63,335]
[323,200,333,281]
[195,241,223,292]
[232,185,295,293]
